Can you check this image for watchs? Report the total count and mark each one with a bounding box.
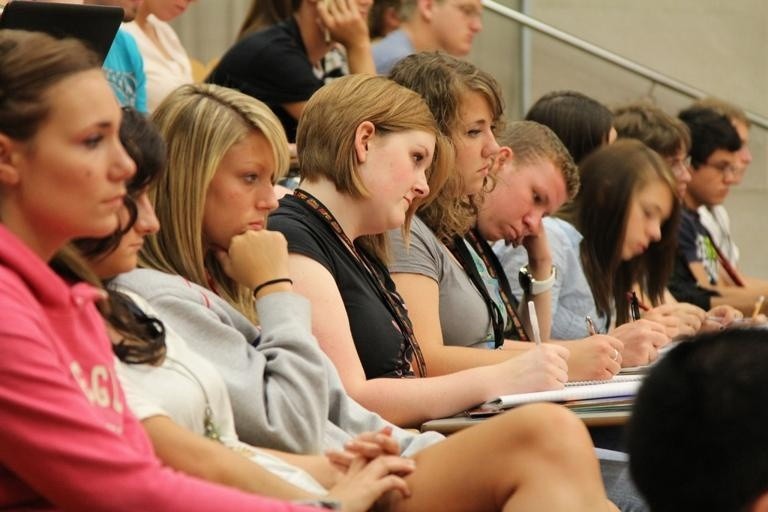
[518,262,557,296]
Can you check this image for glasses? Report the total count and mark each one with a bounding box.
[671,155,693,173]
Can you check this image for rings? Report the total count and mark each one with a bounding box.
[614,349,619,361]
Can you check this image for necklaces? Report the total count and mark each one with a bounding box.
[134,351,255,458]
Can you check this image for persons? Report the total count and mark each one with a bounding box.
[521,90,745,340]
[492,140,679,348]
[387,51,624,380]
[49,104,404,501]
[121,0,200,116]
[677,106,768,297]
[612,102,768,317]
[134,83,651,511]
[693,95,767,288]
[468,120,668,367]
[368,0,402,43]
[206,0,378,159]
[236,0,299,45]
[265,73,631,450]
[372,0,483,78]
[83,0,147,116]
[2,29,621,512]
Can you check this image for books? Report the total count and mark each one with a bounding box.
[465,372,646,417]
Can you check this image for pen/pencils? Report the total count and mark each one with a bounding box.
[527,301,541,345]
[585,315,595,335]
[626,291,648,311]
[631,291,640,320]
[752,296,765,318]
